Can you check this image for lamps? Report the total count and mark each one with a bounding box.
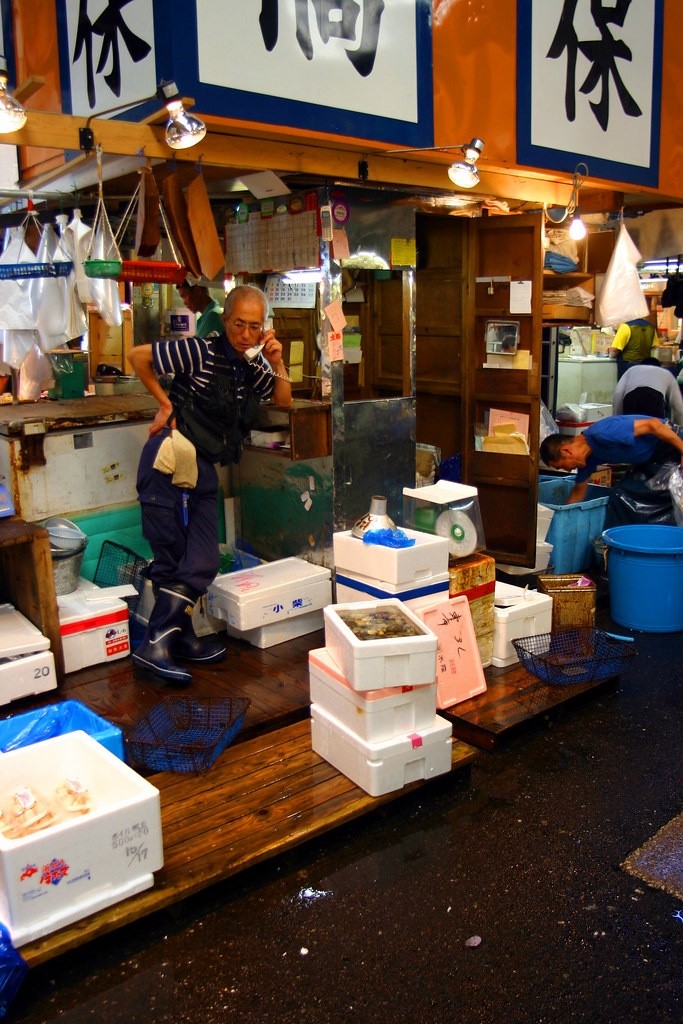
[0,54,27,134]
[78,80,208,149]
[357,137,484,188]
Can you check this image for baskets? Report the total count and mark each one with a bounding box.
[511,625,637,686]
[117,261,186,284]
[0,262,72,280]
[93,539,148,624]
[124,694,251,774]
[46,527,87,550]
[83,260,120,278]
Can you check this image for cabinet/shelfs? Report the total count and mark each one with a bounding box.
[483,207,594,321]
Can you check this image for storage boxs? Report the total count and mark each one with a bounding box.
[542,304,591,321]
[0,478,616,952]
[555,403,613,437]
[640,278,668,293]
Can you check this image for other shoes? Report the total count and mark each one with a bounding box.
[594,583,610,615]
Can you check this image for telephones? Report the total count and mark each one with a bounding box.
[244,317,274,362]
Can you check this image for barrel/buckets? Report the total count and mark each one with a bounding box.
[602,525,683,633]
[52,543,86,597]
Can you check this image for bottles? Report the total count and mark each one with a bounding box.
[170,307,195,335]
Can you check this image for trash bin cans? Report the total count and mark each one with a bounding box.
[539,573,596,635]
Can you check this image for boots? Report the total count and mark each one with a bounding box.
[151,582,226,664]
[132,588,198,684]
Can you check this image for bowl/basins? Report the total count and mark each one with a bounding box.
[83,259,121,278]
[49,528,86,548]
[43,516,82,551]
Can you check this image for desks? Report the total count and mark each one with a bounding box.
[0,514,66,688]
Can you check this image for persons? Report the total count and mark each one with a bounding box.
[614,356,683,426]
[609,318,654,376]
[502,336,515,354]
[128,286,293,677]
[540,415,683,503]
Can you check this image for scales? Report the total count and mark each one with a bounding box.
[42,350,86,399]
[402,478,487,560]
